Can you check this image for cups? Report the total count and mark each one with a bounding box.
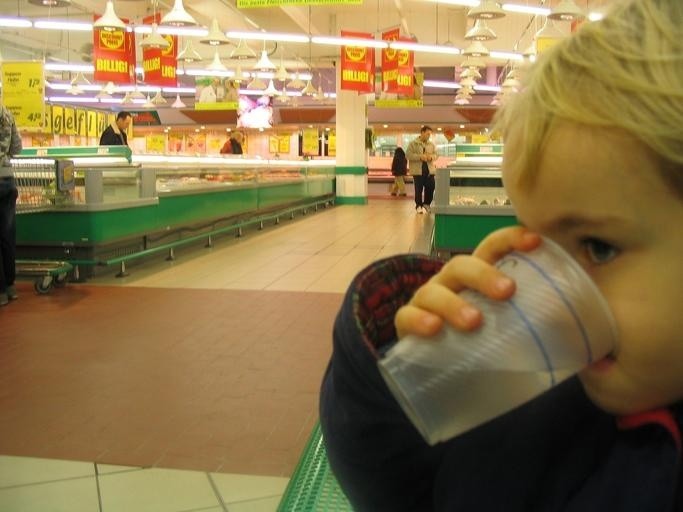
[378,235,622,447]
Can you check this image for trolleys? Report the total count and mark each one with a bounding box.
[0,156,76,295]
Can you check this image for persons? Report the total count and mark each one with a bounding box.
[218,131,245,155]
[405,126,439,214]
[389,144,408,198]
[197,78,219,105]
[221,78,237,102]
[98,112,131,146]
[317,1,681,512]
[0,102,23,307]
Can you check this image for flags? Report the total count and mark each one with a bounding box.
[92,13,132,84]
[157,22,178,88]
[112,21,138,87]
[141,12,162,83]
[398,32,417,96]
[339,29,374,93]
[378,25,401,94]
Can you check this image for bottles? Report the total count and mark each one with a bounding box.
[199,79,227,103]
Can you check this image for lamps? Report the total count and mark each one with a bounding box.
[453,1,585,106]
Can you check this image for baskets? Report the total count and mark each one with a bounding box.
[11,158,75,218]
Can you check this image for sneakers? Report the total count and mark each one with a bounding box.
[416,205,423,214]
[422,201,432,214]
[0,283,19,306]
[390,191,407,197]
